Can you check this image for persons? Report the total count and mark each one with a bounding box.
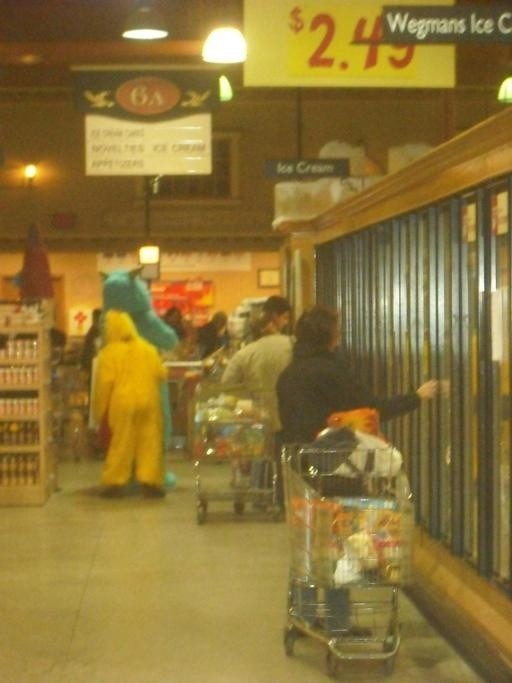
[262,295,293,332]
[274,306,439,638]
[221,317,298,444]
[165,304,229,360]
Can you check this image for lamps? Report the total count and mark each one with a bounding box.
[20,151,45,183]
[137,177,161,268]
[496,76,511,106]
[119,2,170,43]
[200,24,249,66]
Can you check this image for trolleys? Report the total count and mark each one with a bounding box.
[282,444,414,677]
[193,406,278,525]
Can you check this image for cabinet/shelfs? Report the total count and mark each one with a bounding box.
[0,298,53,509]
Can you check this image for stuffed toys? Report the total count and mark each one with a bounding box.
[97,264,180,495]
[88,309,170,500]
